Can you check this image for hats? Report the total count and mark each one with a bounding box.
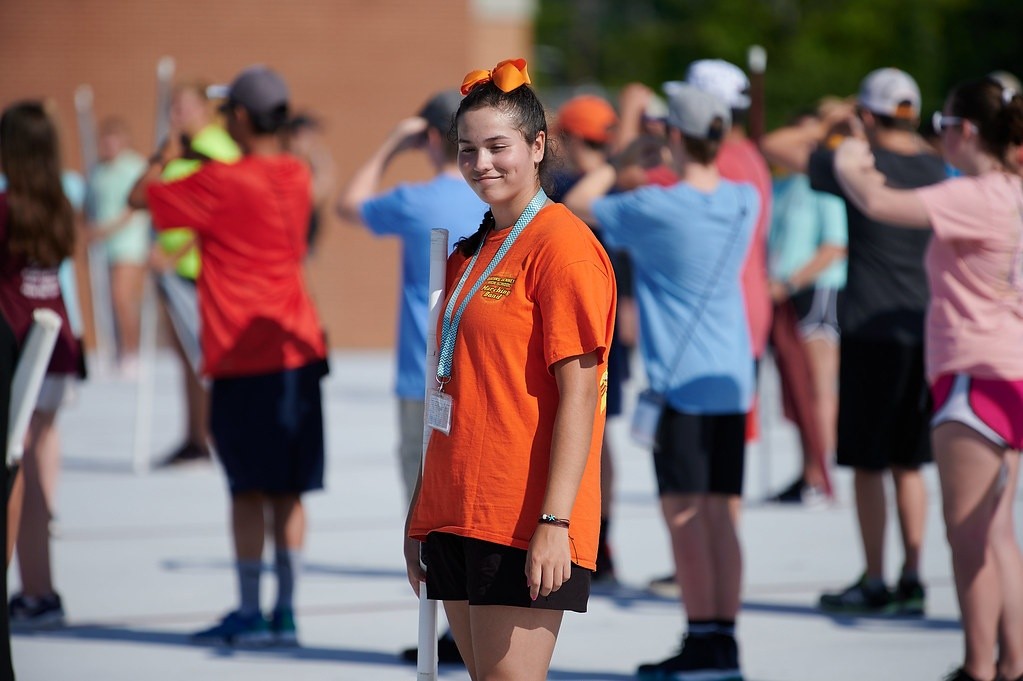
[661,58,753,110]
[644,84,733,140]
[857,67,922,119]
[422,89,469,139]
[217,68,286,126]
[546,95,618,144]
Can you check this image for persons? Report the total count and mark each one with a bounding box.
[58,76,325,468]
[403,59,619,681]
[127,72,331,644]
[836,70,1023,681]
[564,93,759,681]
[0,101,77,632]
[337,60,966,671]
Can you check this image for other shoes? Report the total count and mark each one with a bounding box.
[639,627,743,680]
[399,628,469,670]
[764,476,835,508]
[7,588,64,628]
[162,440,208,467]
[192,606,299,647]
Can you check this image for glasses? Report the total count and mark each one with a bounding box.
[932,111,978,137]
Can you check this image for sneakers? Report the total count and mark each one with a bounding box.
[891,576,926,618]
[821,570,896,617]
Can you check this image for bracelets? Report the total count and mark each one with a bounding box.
[538,514,569,529]
[150,155,166,169]
